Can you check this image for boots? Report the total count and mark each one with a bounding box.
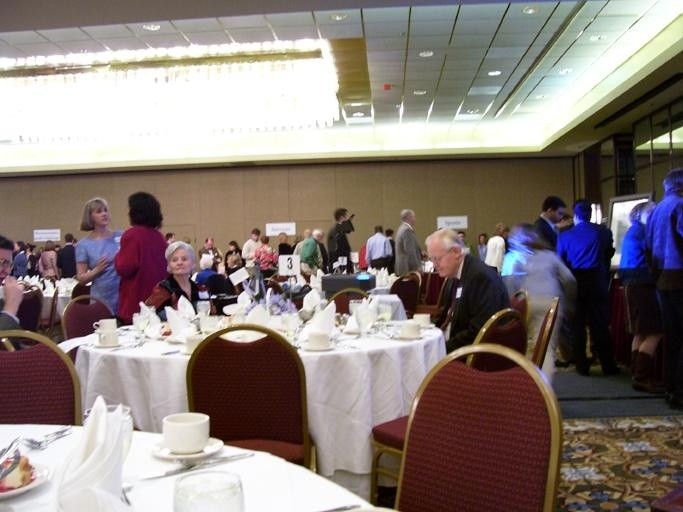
[633,351,666,393]
[630,348,668,389]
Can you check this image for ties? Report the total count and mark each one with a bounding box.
[439,279,458,330]
[555,226,559,236]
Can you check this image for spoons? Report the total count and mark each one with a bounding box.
[21,427,72,450]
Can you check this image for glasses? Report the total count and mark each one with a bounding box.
[1,258,14,270]
[428,247,454,262]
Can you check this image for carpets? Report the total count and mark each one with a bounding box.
[555,415,681,512]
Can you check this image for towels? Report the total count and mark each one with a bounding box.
[177,295,196,316]
[133,301,163,339]
[366,266,388,286]
[297,288,328,317]
[52,395,125,511]
[166,307,199,343]
[310,268,325,289]
[344,300,378,335]
[304,301,338,351]
[17,275,80,296]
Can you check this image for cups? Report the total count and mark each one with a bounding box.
[99,331,117,344]
[132,312,150,342]
[355,304,377,348]
[93,318,117,333]
[308,331,335,347]
[196,300,211,318]
[401,320,423,335]
[163,412,211,453]
[187,336,203,351]
[84,404,134,468]
[377,302,393,340]
[348,298,363,314]
[173,469,244,512]
[413,313,430,327]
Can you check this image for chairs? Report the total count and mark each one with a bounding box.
[389,272,420,324]
[530,295,562,369]
[422,269,460,323]
[327,287,369,320]
[394,343,565,512]
[60,296,115,366]
[1,328,82,427]
[366,308,528,509]
[185,324,321,474]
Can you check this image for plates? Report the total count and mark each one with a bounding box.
[303,346,336,352]
[0,457,54,499]
[395,335,427,339]
[149,437,224,461]
[93,343,121,348]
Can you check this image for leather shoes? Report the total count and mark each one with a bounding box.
[669,392,683,410]
[665,386,676,402]
[577,361,590,375]
[603,362,619,376]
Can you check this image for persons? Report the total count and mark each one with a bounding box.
[183,235,193,245]
[425,229,512,365]
[294,230,313,256]
[458,230,478,253]
[224,242,246,277]
[75,198,125,328]
[557,199,624,377]
[485,223,512,277]
[144,241,219,322]
[199,238,222,274]
[358,244,370,272]
[645,167,683,411]
[329,208,354,274]
[384,228,396,273]
[395,209,424,276]
[534,195,566,255]
[364,226,392,275]
[278,233,294,254]
[290,236,303,256]
[56,232,77,281]
[299,230,326,268]
[115,192,178,328]
[477,232,489,272]
[554,214,576,367]
[501,222,577,386]
[318,242,329,276]
[190,257,221,301]
[255,237,280,280]
[619,202,667,394]
[0,235,25,351]
[39,240,62,282]
[25,243,37,278]
[11,241,28,280]
[242,230,263,291]
[164,232,174,251]
